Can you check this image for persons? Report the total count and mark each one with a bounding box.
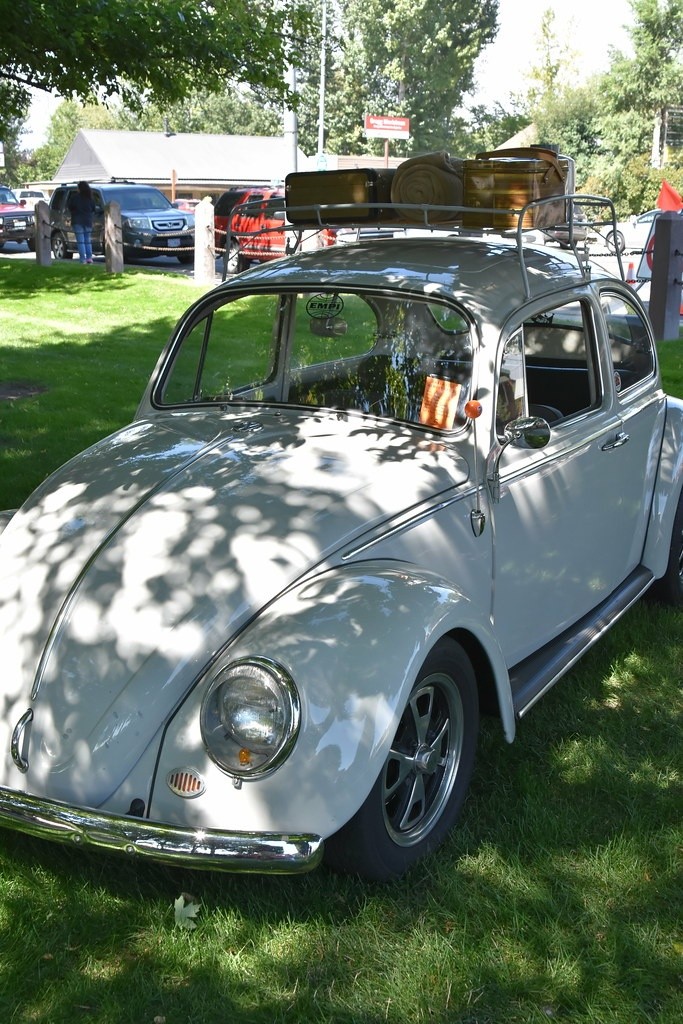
[68,181,95,264]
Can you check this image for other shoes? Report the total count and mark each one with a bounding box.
[82,260,87,264]
[87,258,93,263]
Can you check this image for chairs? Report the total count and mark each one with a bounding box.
[527,404,563,428]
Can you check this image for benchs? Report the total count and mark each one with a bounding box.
[357,354,636,418]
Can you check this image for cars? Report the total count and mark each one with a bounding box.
[597,208,670,255]
[0,196,681,881]
[6,188,52,213]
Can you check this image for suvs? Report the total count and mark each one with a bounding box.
[214,183,347,276]
[46,177,197,262]
[0,185,38,253]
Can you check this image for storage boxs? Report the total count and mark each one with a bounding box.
[463,147,574,229]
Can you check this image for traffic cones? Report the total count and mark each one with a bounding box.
[626,261,638,292]
